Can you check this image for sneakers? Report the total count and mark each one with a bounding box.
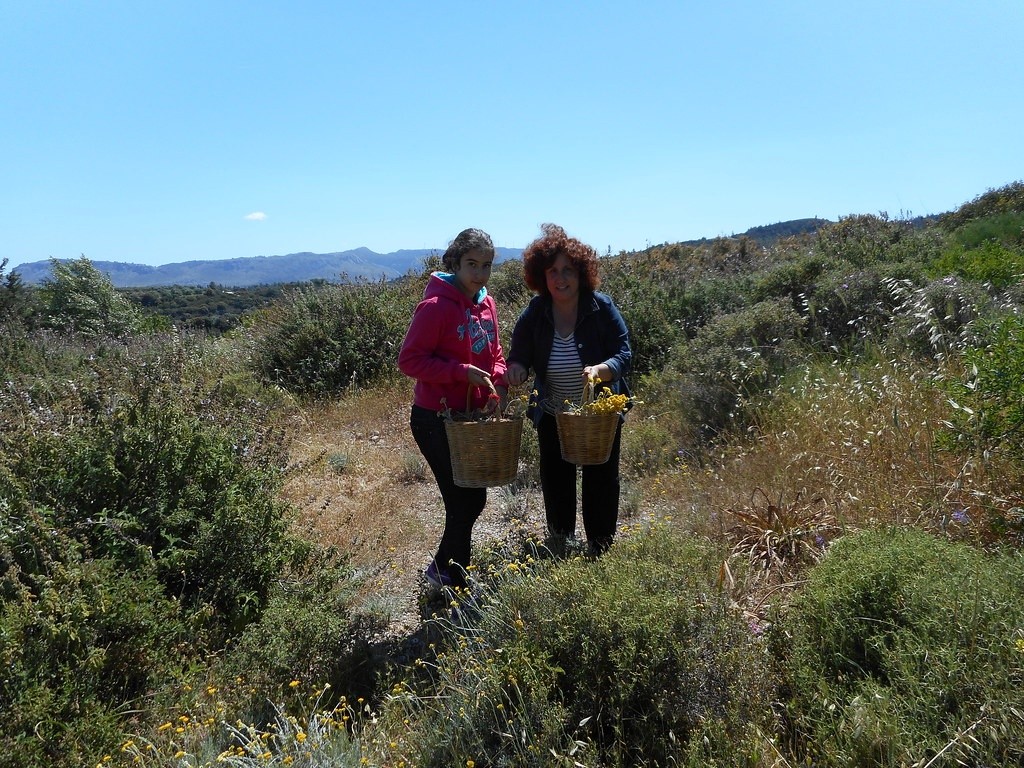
[424,564,452,592]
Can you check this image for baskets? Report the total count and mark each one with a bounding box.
[444,376,522,487]
[555,373,621,465]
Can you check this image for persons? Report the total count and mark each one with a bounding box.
[505,223,635,562]
[399,228,510,590]
